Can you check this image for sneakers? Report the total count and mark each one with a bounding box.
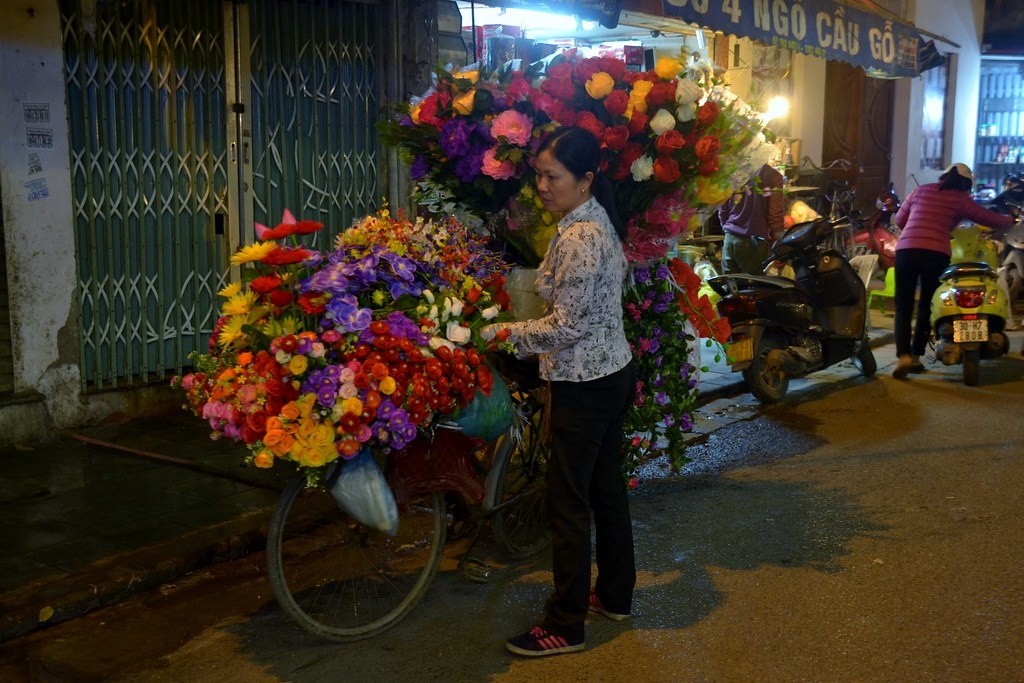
[587,587,630,621]
[504,622,586,656]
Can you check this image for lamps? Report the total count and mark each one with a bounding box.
[545,0,622,30]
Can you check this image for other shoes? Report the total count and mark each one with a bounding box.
[891,356,912,380]
[910,361,925,373]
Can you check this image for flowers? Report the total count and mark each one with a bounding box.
[373,57,780,489]
[170,197,522,492]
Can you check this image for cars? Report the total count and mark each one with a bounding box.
[973,189,996,204]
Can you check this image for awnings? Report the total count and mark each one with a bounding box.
[458,0,624,29]
[661,0,961,80]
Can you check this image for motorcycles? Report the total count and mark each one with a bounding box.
[706,214,877,405]
[927,222,1010,386]
[845,180,903,270]
[984,171,1024,304]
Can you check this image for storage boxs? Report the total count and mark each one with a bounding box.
[774,136,801,168]
[464,24,647,73]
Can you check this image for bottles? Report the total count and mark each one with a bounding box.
[997,145,1024,164]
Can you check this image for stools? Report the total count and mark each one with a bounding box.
[822,222,853,257]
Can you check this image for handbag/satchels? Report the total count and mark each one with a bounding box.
[328,451,400,538]
[451,351,514,442]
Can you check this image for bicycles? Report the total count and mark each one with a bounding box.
[264,350,559,645]
[800,155,859,222]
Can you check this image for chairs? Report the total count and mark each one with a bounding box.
[849,255,880,289]
[867,266,896,315]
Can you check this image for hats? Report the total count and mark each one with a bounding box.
[943,162,973,179]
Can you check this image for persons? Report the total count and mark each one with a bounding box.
[718,163,784,292]
[892,163,1013,379]
[480,125,637,656]
